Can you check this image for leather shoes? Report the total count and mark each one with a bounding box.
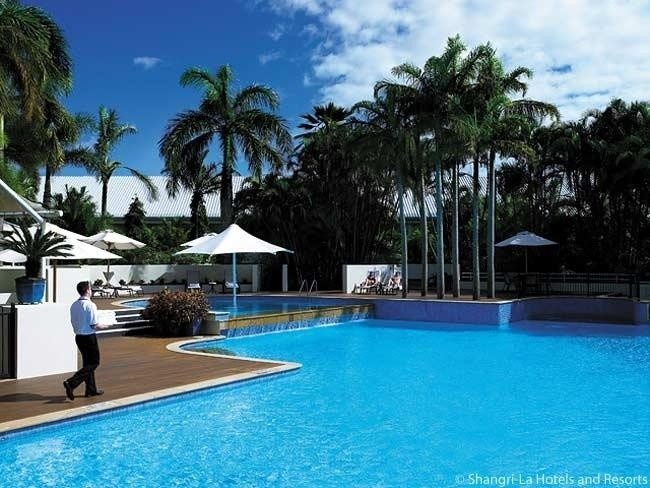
[63,379,74,402]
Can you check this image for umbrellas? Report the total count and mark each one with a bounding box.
[3,222,92,302]
[179,231,226,282]
[0,232,123,302]
[494,231,560,272]
[83,228,148,288]
[173,223,295,307]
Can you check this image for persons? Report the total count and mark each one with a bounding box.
[388,271,401,287]
[427,272,437,292]
[63,282,113,400]
[444,272,449,294]
[361,272,375,287]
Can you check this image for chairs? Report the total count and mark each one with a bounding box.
[501,266,542,299]
[222,269,240,294]
[351,271,403,295]
[90,283,145,299]
[185,270,202,294]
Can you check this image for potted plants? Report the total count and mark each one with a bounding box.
[0,218,75,303]
[138,286,210,338]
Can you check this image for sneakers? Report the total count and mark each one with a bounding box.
[84,388,105,398]
